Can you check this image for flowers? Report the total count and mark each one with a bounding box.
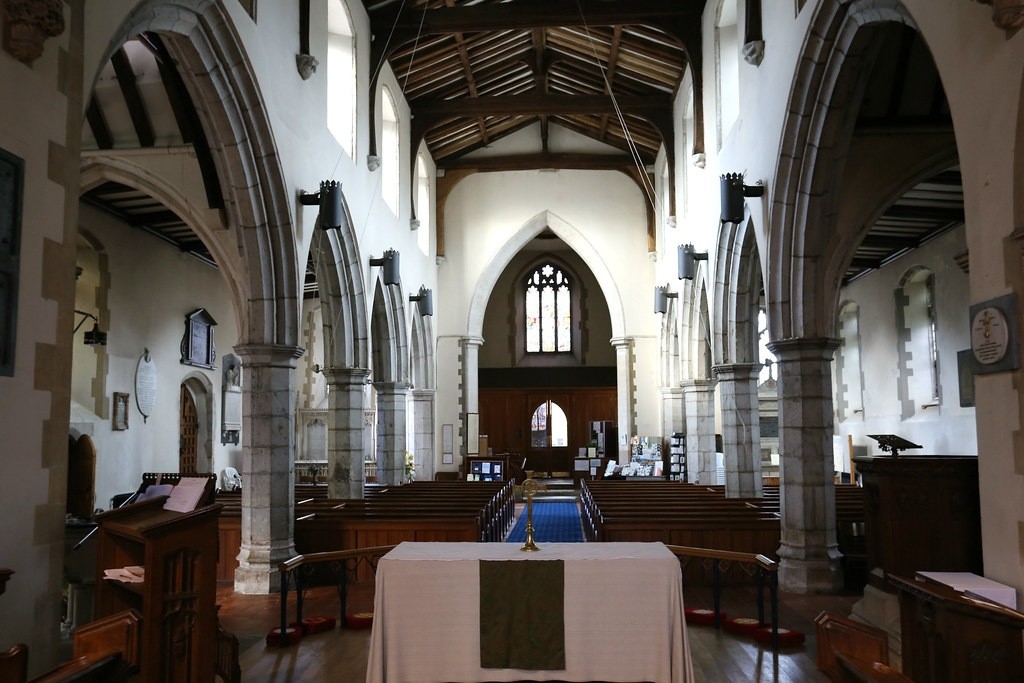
[406,451,413,476]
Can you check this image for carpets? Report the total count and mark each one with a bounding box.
[505,502,584,542]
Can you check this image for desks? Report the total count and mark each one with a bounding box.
[851,455,983,574]
[462,453,510,480]
[381,541,681,683]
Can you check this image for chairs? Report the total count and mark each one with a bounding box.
[223,467,242,491]
[435,472,460,481]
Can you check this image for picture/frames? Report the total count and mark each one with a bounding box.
[113,392,130,430]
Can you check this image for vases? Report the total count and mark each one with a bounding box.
[406,476,411,484]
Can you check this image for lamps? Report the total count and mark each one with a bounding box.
[370,250,400,286]
[678,244,708,280]
[74,309,107,347]
[720,173,765,225]
[299,180,342,231]
[409,287,433,316]
[654,285,678,314]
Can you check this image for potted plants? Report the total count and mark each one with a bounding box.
[307,463,328,485]
